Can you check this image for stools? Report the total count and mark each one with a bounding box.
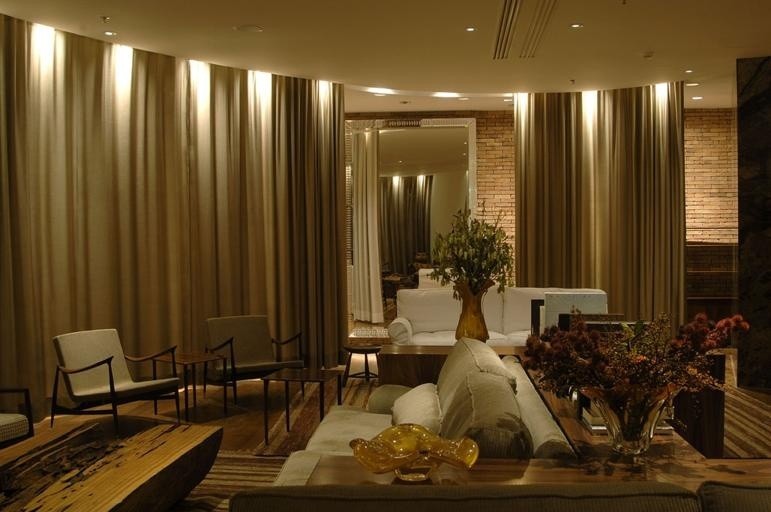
[344,342,381,385]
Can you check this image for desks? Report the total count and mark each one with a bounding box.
[519,353,708,465]
[0,410,223,512]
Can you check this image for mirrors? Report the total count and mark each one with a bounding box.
[342,118,477,339]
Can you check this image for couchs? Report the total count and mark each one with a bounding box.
[228,478,767,511]
[387,285,607,347]
[298,343,579,463]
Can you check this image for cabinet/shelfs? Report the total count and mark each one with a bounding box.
[683,240,740,347]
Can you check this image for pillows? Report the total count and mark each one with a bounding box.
[391,381,442,436]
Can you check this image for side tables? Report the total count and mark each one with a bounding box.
[155,352,227,421]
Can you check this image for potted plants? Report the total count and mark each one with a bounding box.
[427,199,516,344]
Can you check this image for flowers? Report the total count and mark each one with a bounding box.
[522,304,748,440]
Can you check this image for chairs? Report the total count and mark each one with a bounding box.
[50,328,181,424]
[204,315,305,411]
[0,387,32,448]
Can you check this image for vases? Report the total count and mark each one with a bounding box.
[583,385,685,454]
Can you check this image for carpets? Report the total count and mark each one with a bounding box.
[254,361,380,458]
